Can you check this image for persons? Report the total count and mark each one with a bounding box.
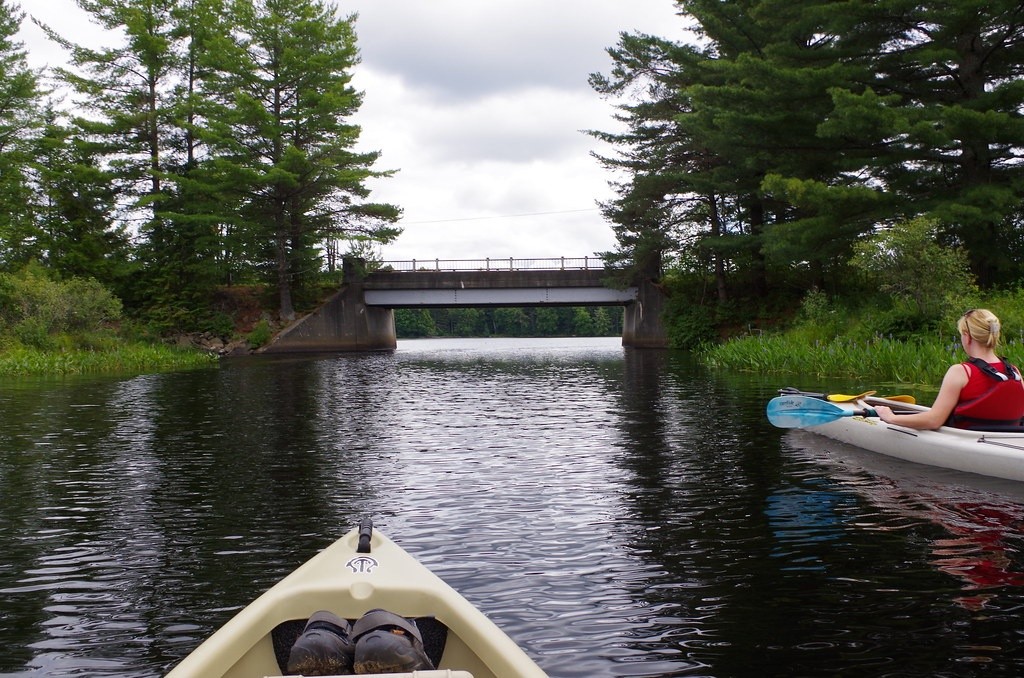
[874,309,1024,433]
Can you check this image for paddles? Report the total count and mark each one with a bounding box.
[881,392,918,404]
[765,394,927,430]
[775,387,879,403]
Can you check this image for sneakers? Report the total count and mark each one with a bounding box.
[286,610,355,676]
[348,608,436,673]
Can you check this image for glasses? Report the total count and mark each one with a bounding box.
[963,310,975,339]
